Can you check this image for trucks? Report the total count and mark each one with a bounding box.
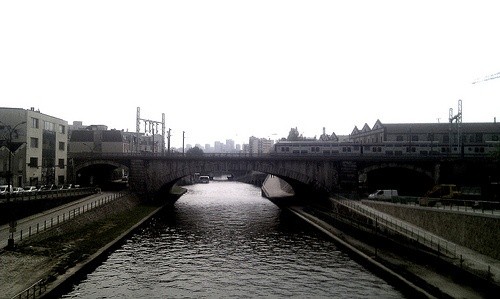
[369,189,397,202]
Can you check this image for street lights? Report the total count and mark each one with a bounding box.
[183,131,186,155]
[166,128,171,153]
[0,121,26,198]
[84,143,102,185]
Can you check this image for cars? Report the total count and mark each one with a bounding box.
[0,184,79,195]
